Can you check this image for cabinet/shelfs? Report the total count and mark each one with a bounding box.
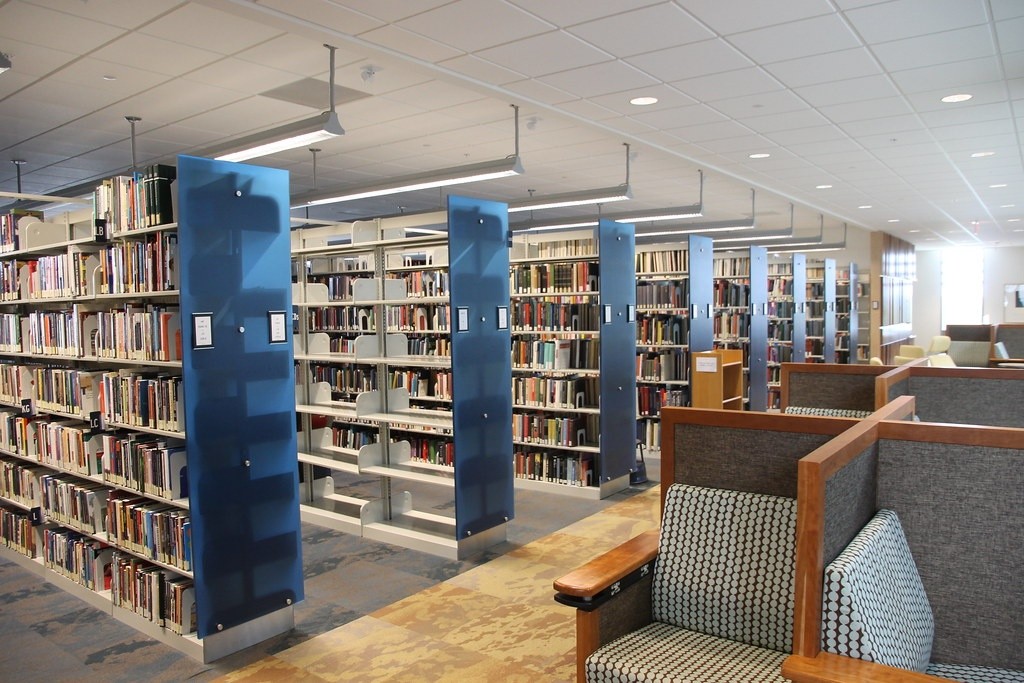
[0,155,876,666]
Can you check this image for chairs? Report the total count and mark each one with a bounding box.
[553,325,1024,683]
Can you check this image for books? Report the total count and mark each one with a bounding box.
[0,164,197,637]
[767,263,793,411]
[291,270,453,469]
[806,268,870,364]
[713,257,750,398]
[509,240,600,489]
[636,250,690,453]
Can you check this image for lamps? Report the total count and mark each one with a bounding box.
[290,156,521,212]
[511,206,702,233]
[717,228,794,246]
[771,242,847,254]
[505,186,630,214]
[718,237,823,252]
[633,219,754,238]
[209,111,345,162]
